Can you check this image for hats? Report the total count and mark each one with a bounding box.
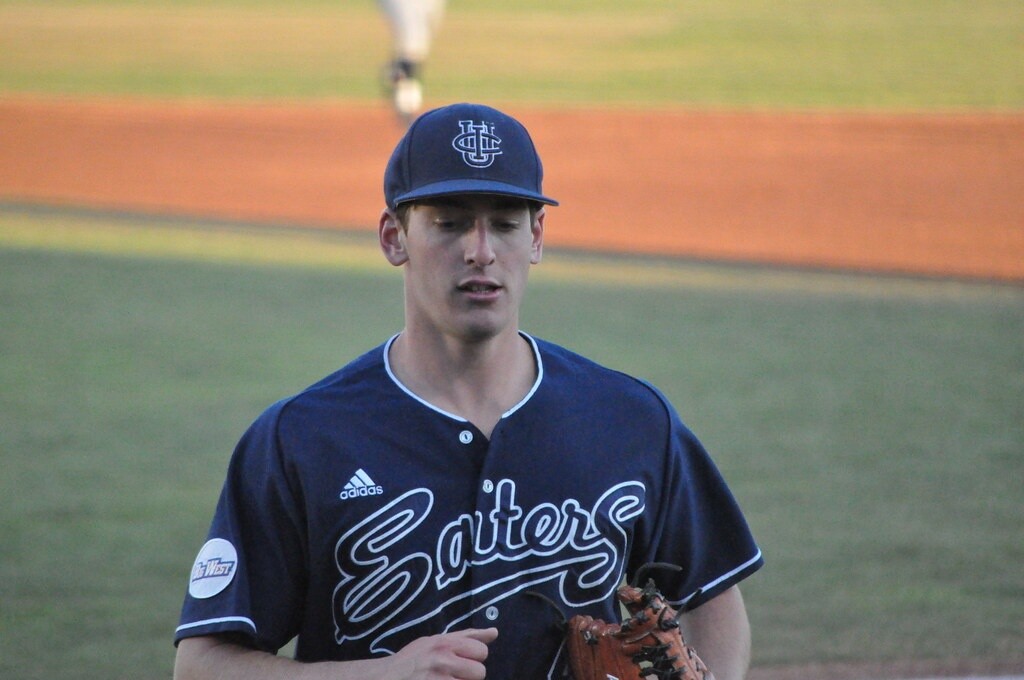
[383,102,560,207]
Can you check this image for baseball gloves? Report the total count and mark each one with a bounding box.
[567,584,715,680]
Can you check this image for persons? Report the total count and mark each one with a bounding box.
[375,0,445,125]
[173,103,765,680]
